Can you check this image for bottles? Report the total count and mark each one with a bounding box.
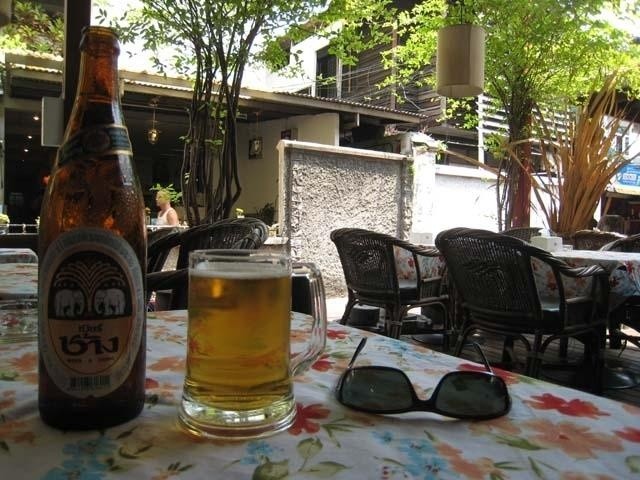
[35,25,149,434]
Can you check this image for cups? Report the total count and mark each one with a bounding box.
[173,247,329,442]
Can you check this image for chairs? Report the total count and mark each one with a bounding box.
[498,228,543,244]
[569,229,627,249]
[597,214,625,234]
[144,217,269,310]
[593,234,640,369]
[146,226,178,310]
[435,228,606,381]
[330,228,451,352]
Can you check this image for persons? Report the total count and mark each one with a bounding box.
[154,189,180,226]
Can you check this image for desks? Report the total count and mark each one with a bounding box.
[1,263,40,311]
[0,311,640,479]
[1,247,38,262]
[394,245,639,341]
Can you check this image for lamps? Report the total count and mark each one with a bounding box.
[435,2,486,99]
[252,112,262,155]
[147,99,160,146]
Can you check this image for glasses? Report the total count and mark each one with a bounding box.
[337,336,510,417]
